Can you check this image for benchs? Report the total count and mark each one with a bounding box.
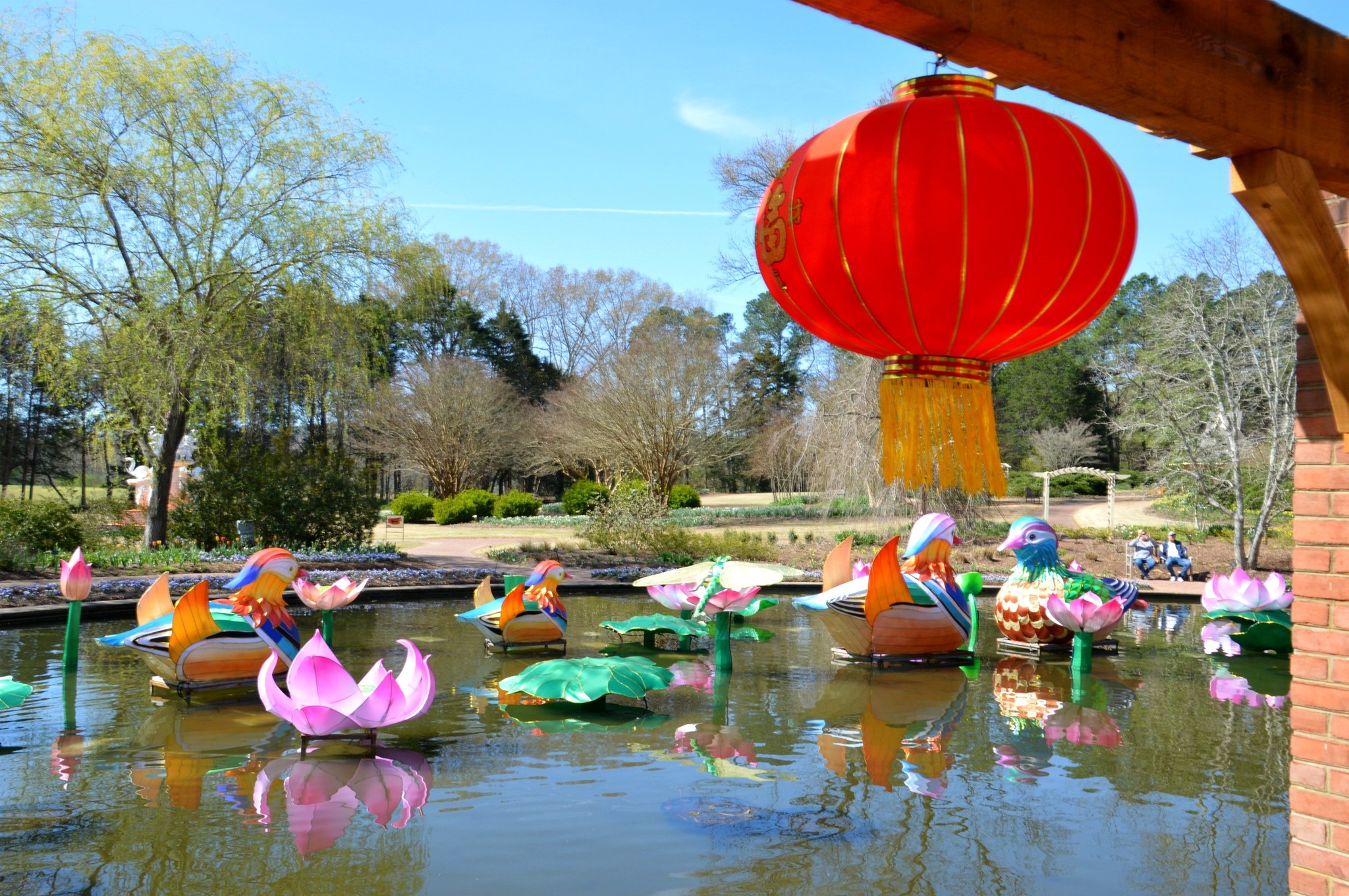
[1125,543,1193,581]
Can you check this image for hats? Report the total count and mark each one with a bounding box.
[1168,531,1176,535]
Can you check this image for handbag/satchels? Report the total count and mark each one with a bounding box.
[1152,554,1160,563]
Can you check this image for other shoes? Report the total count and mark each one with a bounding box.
[1170,576,1177,581]
[1146,575,1151,580]
[1178,577,1184,582]
[1140,574,1145,580]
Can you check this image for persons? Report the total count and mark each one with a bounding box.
[1159,531,1191,582]
[1129,529,1159,580]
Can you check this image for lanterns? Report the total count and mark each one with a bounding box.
[754,70,1139,497]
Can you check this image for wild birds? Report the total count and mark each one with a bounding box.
[791,512,972,656]
[996,515,1139,643]
[94,547,311,680]
[455,559,575,642]
[125,456,153,478]
